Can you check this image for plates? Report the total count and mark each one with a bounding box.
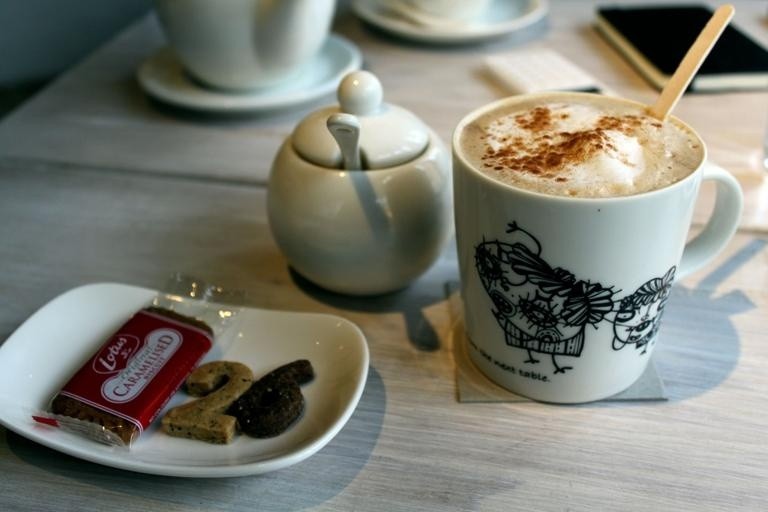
[135,30,363,116]
[0,282,371,480]
[351,0,550,44]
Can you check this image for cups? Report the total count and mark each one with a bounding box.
[156,1,336,91]
[450,90,743,406]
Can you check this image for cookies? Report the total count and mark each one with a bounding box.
[52,305,316,448]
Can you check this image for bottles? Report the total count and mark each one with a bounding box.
[268,71,454,296]
